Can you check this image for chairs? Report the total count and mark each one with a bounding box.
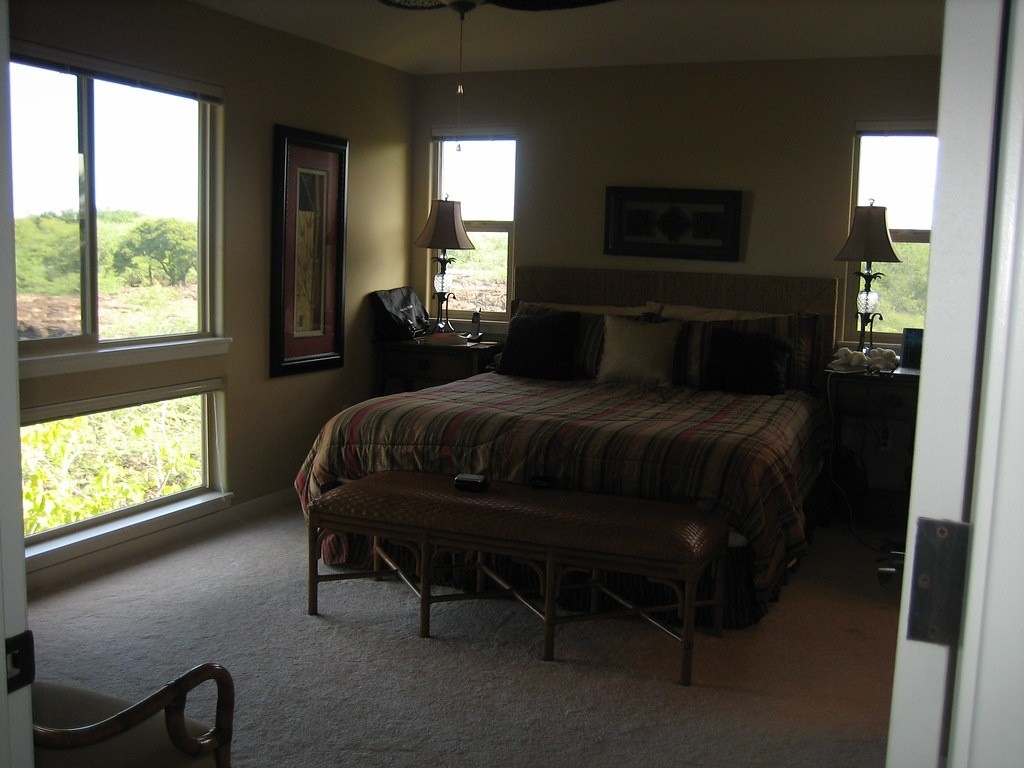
[30,663,234,768]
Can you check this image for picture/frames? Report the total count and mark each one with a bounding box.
[603,186,745,262]
[268,122,351,377]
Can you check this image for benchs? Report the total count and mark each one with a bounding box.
[307,471,732,686]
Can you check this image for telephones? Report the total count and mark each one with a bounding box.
[466,307,484,342]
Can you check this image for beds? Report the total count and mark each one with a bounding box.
[293,256,839,628]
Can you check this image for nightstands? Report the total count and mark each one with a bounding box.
[823,363,920,495]
[369,338,505,398]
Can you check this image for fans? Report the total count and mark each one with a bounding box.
[373,0,618,19]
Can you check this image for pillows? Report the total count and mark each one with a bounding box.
[493,295,825,396]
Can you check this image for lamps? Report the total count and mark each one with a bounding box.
[834,205,901,352]
[411,199,476,333]
